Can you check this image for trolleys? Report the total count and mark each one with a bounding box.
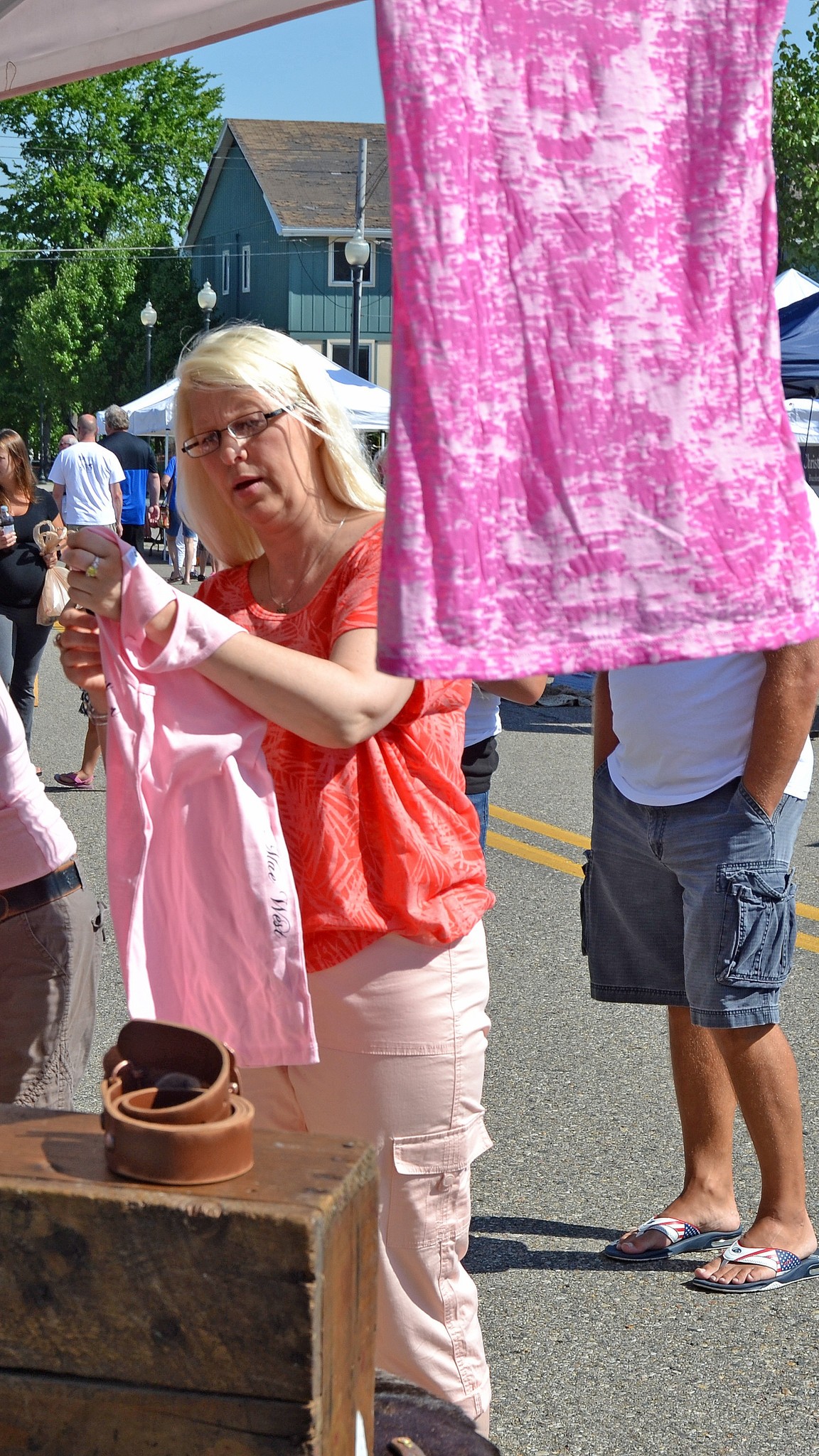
[150,493,168,555]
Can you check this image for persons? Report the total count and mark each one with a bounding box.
[0,429,67,775]
[59,434,78,451]
[461,673,550,854]
[0,677,103,1106]
[161,457,196,584]
[97,404,160,560]
[60,326,491,1445]
[170,522,223,582]
[54,685,101,789]
[48,414,126,538]
[581,477,818,1297]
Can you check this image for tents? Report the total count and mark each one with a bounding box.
[97,343,391,566]
[773,268,819,310]
[785,398,819,447]
[778,292,819,400]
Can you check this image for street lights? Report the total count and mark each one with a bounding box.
[141,298,157,393]
[198,277,216,331]
[344,222,371,376]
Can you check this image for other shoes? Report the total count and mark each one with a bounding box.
[171,571,183,580]
[209,572,215,576]
[197,575,207,581]
[189,570,198,580]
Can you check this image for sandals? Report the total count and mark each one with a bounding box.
[54,772,94,789]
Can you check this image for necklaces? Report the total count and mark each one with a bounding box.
[268,506,352,613]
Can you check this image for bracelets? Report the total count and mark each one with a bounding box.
[56,545,61,560]
[87,708,108,727]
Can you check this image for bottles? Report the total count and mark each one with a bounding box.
[0,505,17,553]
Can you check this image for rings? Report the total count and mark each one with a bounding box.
[54,633,62,648]
[86,557,99,577]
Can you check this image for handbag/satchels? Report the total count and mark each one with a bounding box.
[33,521,84,626]
[157,506,170,529]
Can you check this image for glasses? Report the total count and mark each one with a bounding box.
[181,401,305,458]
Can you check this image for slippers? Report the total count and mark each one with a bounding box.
[181,580,191,585]
[603,1214,742,1261]
[167,576,180,583]
[690,1238,819,1293]
